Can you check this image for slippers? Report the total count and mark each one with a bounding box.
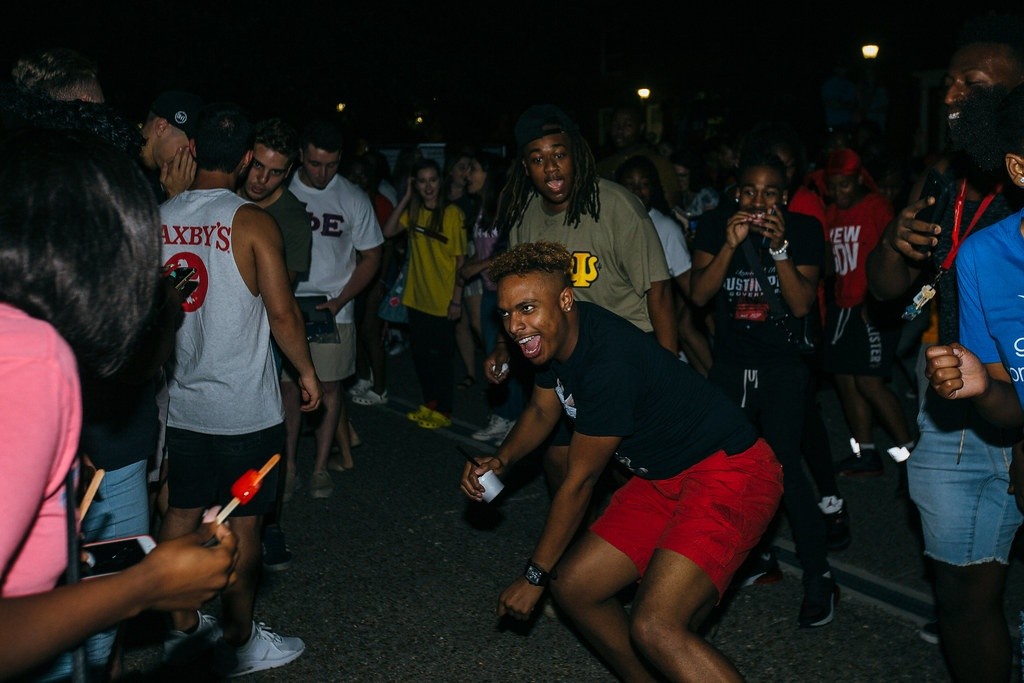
[406,405,452,428]
[326,458,354,472]
[332,438,361,455]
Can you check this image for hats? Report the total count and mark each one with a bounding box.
[515,104,578,150]
[824,148,861,177]
[150,92,202,131]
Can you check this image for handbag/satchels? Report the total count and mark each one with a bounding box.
[799,305,822,355]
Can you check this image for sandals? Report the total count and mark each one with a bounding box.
[457,375,477,392]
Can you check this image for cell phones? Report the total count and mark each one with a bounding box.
[903,169,950,268]
[78,536,158,581]
[761,209,777,249]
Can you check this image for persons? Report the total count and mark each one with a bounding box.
[1,103,240,682]
[0,50,158,683]
[138,87,942,625]
[863,32,1024,683]
[154,108,328,677]
[459,240,789,683]
[924,80,1023,511]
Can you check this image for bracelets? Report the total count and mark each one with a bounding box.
[450,300,463,306]
[772,251,788,261]
[496,340,508,346]
[769,240,788,255]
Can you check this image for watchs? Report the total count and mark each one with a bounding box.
[524,558,558,590]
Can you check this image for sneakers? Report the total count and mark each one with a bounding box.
[725,548,780,591]
[161,610,223,661]
[385,336,409,356]
[218,620,306,679]
[282,475,305,502]
[886,446,915,500]
[817,495,851,551]
[348,380,373,396]
[836,437,884,478]
[796,566,838,628]
[919,620,939,645]
[472,415,517,448]
[353,390,388,405]
[309,468,332,499]
[260,523,293,570]
